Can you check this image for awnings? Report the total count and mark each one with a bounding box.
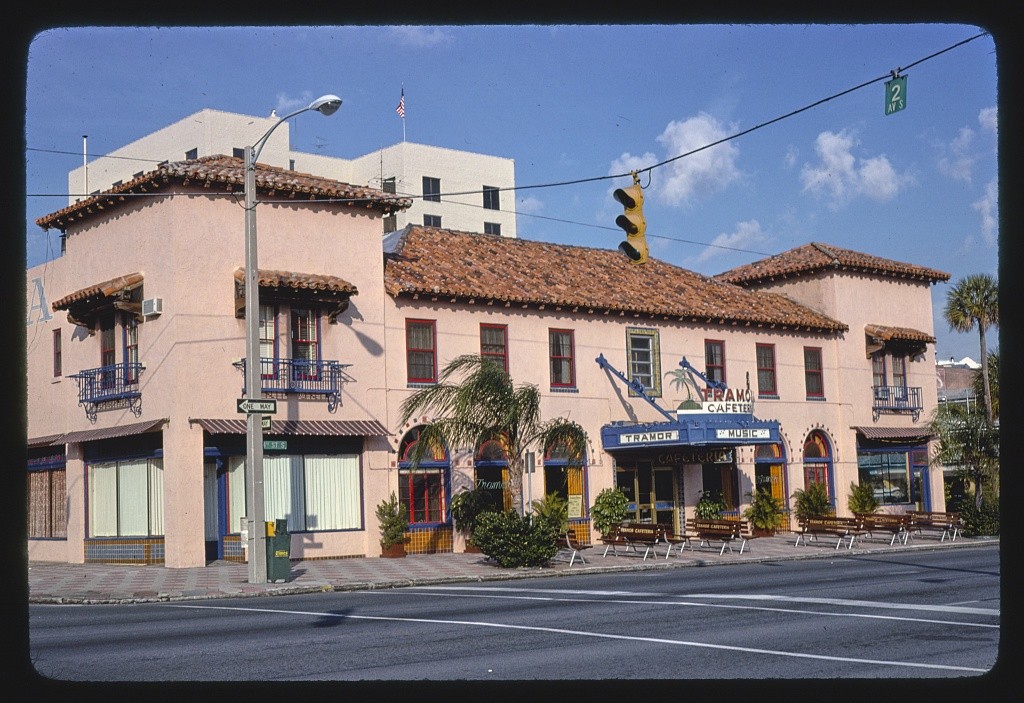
[199,418,390,437]
[859,426,935,438]
[26,433,63,447]
[48,417,165,445]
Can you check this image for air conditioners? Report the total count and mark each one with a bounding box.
[141,298,162,316]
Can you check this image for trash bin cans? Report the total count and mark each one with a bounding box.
[266,519,290,581]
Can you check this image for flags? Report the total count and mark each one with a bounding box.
[395,86,406,120]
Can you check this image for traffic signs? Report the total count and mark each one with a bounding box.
[260,416,273,431]
[236,398,278,414]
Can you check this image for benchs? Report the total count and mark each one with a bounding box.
[795,512,962,551]
[555,530,593,567]
[601,517,751,556]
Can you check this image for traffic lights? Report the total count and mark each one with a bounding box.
[612,184,652,265]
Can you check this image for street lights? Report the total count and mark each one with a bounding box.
[243,94,342,584]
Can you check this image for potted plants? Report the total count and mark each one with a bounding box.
[696,489,728,540]
[448,485,495,551]
[589,487,629,540]
[376,491,408,556]
[745,488,782,537]
[792,480,831,530]
[849,481,880,527]
